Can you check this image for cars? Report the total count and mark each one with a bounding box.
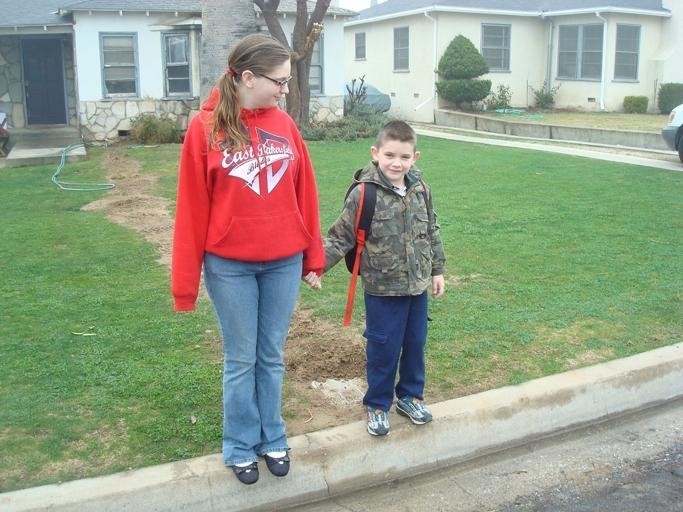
[661,102,683,165]
[0,112,17,157]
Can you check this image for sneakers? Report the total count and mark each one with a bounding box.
[395,394,434,425]
[365,404,391,437]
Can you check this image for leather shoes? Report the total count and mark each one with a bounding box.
[230,461,259,485]
[263,449,290,477]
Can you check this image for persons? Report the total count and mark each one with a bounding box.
[169,31,327,486]
[301,119,448,439]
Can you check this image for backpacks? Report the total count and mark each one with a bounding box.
[344,177,379,276]
